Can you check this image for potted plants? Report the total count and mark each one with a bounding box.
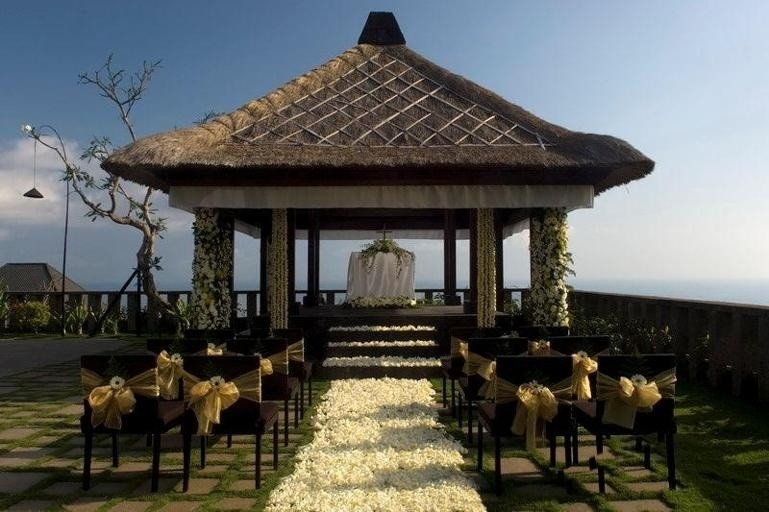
[616,317,763,404]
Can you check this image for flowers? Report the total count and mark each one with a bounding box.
[358,237,416,280]
[344,295,424,311]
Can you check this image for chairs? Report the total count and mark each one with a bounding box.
[79,327,312,499]
[439,326,677,495]
